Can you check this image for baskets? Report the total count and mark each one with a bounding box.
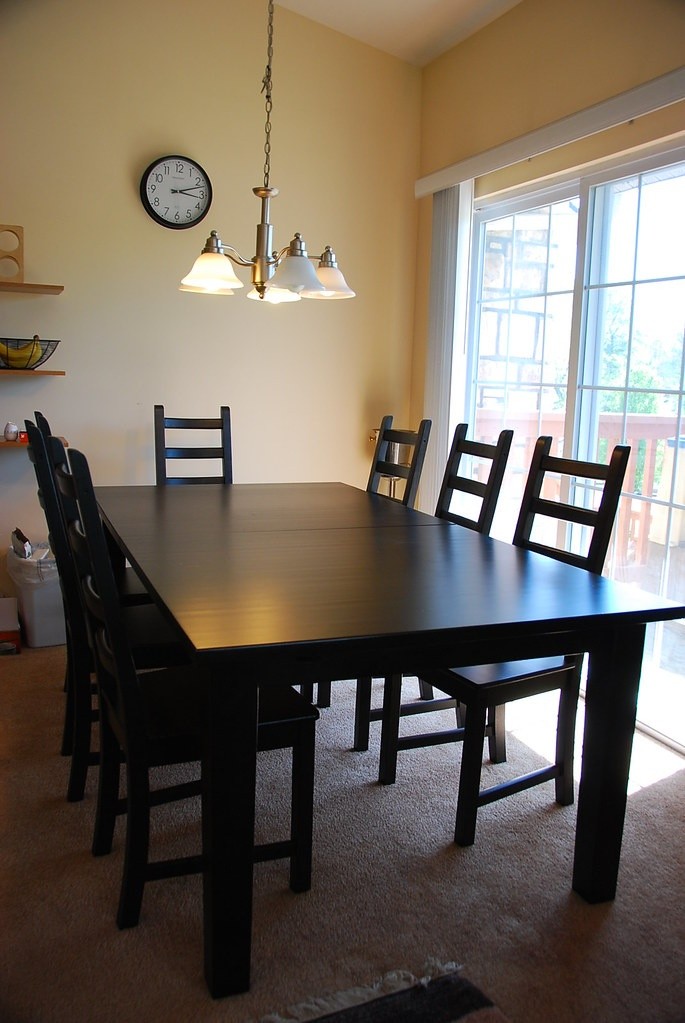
[0,338,61,368]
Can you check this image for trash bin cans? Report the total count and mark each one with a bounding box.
[8,542,66,648]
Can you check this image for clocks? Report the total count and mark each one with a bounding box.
[141,154,216,230]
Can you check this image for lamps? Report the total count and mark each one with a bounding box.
[178,1,357,306]
[370,428,416,498]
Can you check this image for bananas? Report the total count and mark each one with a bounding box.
[0,334,42,368]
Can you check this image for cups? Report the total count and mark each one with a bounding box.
[19,431,29,442]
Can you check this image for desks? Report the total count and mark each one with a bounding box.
[91,481,685,1000]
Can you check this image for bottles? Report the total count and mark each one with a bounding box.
[4,422,19,441]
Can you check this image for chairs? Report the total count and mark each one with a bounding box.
[25,403,632,934]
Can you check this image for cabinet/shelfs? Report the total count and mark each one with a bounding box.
[0,223,69,449]
